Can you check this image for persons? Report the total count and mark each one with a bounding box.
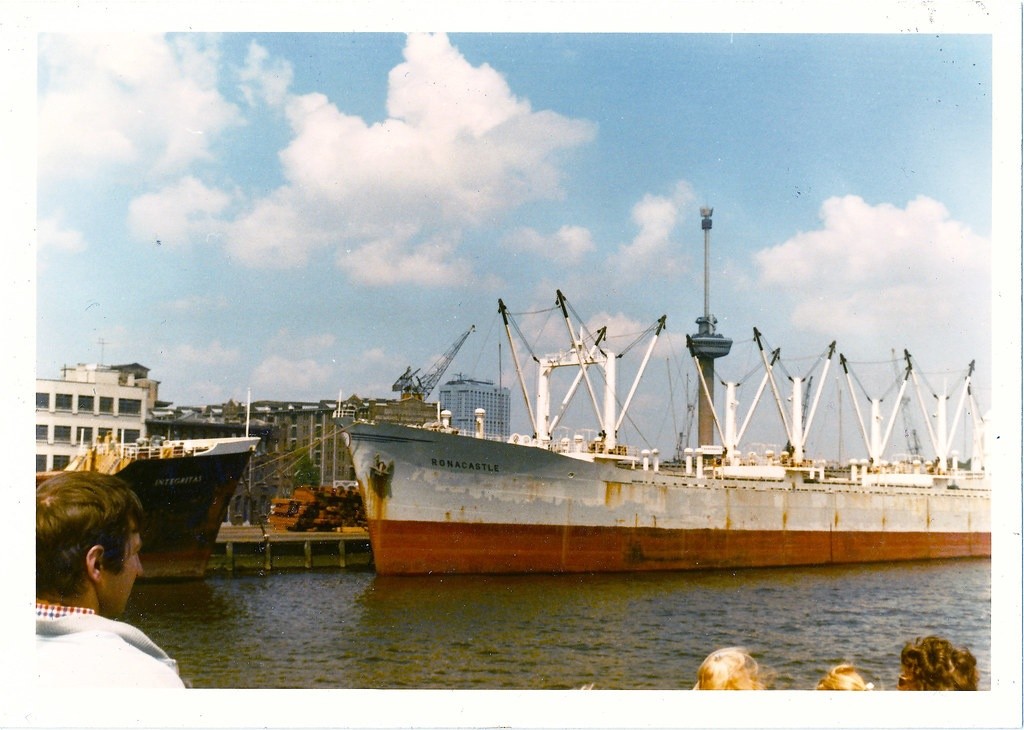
[36,470,186,690]
[816,662,869,691]
[897,634,980,691]
[691,646,769,690]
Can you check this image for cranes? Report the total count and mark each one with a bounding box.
[392,325,475,402]
[890,348,923,455]
[783,376,813,453]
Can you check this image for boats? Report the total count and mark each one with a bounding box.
[330,208,991,578]
[36,431,262,583]
